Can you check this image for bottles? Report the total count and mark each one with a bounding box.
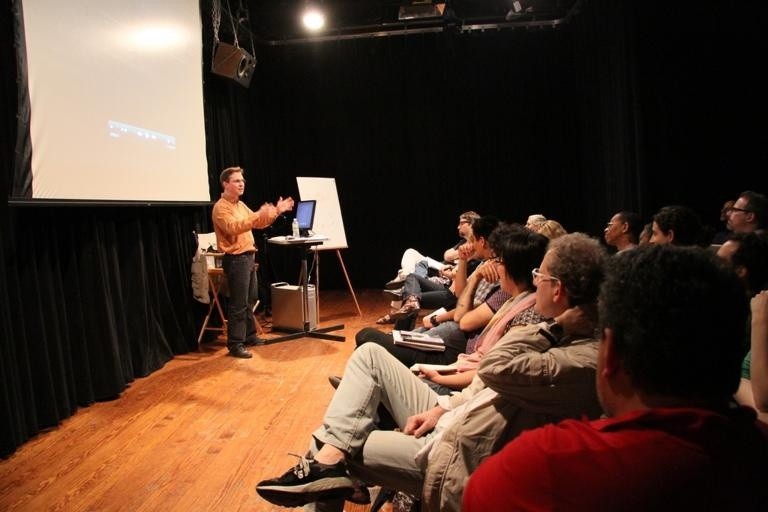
[291,219,300,238]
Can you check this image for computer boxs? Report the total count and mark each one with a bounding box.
[270,280,317,332]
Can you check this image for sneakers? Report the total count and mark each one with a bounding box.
[328,376,342,390]
[229,343,253,358]
[255,449,355,509]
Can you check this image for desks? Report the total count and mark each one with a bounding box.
[265,235,345,343]
[198,267,262,346]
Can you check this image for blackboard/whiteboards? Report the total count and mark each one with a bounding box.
[295,176,350,250]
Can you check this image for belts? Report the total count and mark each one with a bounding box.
[224,250,255,257]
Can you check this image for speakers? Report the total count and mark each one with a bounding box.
[211,41,258,89]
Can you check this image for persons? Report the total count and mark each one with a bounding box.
[257,191,768,510]
[212,166,295,358]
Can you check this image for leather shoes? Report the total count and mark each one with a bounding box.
[376,316,396,324]
[389,299,421,322]
[382,288,403,301]
[386,269,410,288]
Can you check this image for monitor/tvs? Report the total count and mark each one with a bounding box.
[293,200,317,237]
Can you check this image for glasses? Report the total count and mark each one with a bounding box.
[532,267,559,282]
